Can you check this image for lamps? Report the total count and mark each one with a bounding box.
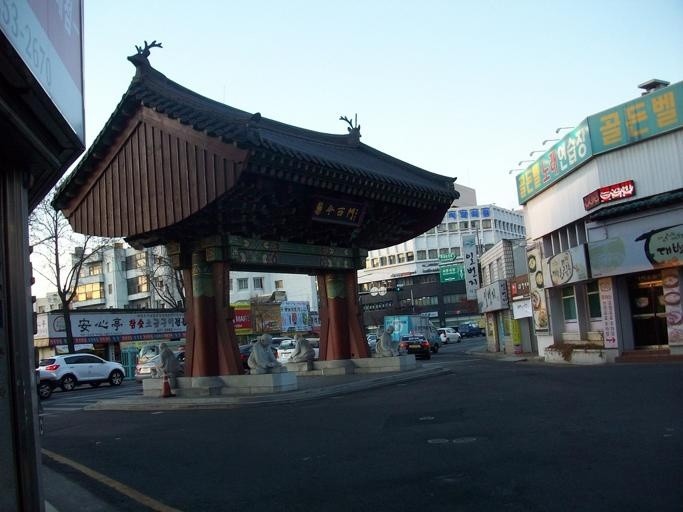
[508,126,575,175]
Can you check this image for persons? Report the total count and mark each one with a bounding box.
[176,345,185,362]
[149,343,183,378]
[288,334,316,362]
[247,334,281,375]
[375,326,397,357]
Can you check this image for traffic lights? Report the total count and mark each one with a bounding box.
[395,286,400,296]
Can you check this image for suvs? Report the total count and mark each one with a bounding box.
[35,354,126,391]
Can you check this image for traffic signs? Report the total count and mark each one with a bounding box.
[439,265,464,283]
[438,252,456,255]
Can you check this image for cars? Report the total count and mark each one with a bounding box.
[237,337,319,369]
[365,314,485,360]
[35,370,56,400]
[133,351,185,384]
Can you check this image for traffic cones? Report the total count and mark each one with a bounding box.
[160,372,176,398]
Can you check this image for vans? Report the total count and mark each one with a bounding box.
[137,341,186,364]
